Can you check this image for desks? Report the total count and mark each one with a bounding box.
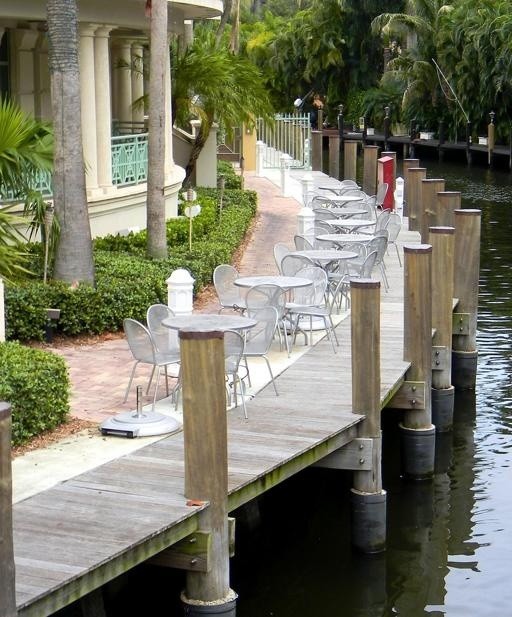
[160,313,259,412]
[234,275,314,351]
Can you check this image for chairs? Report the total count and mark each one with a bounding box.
[245,283,291,358]
[294,266,328,305]
[273,178,402,314]
[287,275,347,356]
[231,305,280,403]
[213,264,247,316]
[120,316,181,414]
[216,330,250,421]
[146,303,183,399]
